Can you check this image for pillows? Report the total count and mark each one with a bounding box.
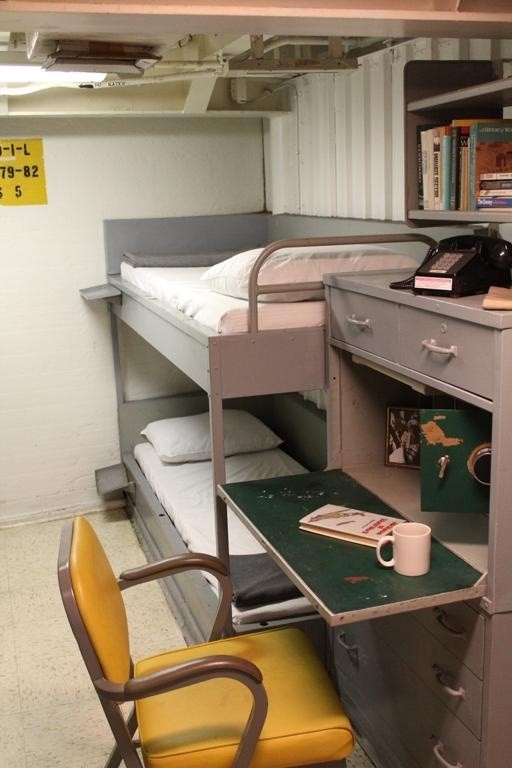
[140,408,285,463]
[199,239,422,303]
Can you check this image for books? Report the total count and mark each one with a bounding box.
[415,120,511,212]
[297,502,409,549]
[482,285,512,310]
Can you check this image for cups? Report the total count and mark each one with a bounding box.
[377,521,432,577]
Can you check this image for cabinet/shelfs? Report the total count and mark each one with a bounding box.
[217,269,512,767]
[403,57,512,228]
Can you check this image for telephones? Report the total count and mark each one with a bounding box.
[390,234,512,298]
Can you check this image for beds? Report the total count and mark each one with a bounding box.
[79,210,437,647]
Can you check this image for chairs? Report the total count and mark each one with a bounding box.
[57,517,354,767]
